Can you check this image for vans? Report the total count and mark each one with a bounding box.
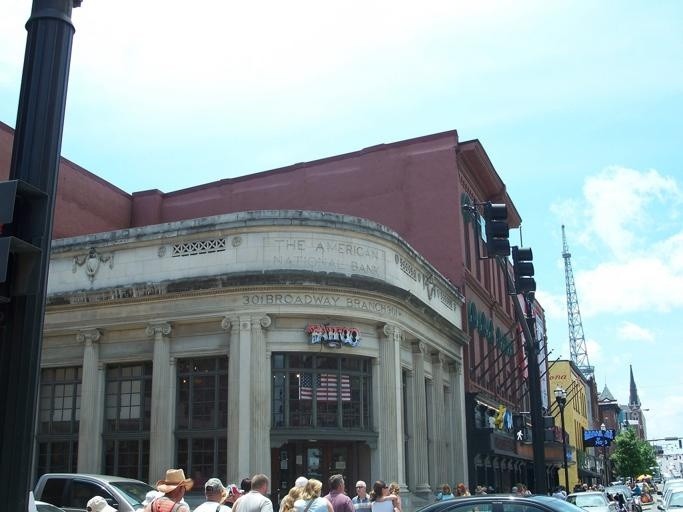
[33,471,191,512]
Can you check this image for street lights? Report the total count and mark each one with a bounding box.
[601,422,609,487]
[553,384,570,494]
[636,409,650,441]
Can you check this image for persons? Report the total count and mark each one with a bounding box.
[510,482,532,495]
[369,480,402,512]
[351,480,371,511]
[550,481,605,500]
[641,482,651,497]
[630,483,640,497]
[134,466,334,512]
[321,473,356,511]
[434,481,495,502]
[374,481,401,512]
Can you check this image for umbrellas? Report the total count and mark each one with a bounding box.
[637,473,652,482]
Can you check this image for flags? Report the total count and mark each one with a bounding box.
[296,369,351,402]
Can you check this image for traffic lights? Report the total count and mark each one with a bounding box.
[543,414,555,444]
[0,177,50,306]
[510,244,538,295]
[512,415,525,442]
[482,200,510,259]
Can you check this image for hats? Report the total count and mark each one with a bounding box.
[86,496,117,512]
[204,478,244,504]
[141,469,193,506]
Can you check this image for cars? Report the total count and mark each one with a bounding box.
[416,494,587,512]
[33,501,67,511]
[566,463,683,512]
